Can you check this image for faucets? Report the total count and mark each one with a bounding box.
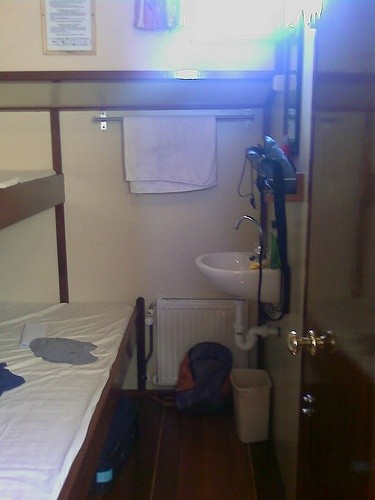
[234,214,268,262]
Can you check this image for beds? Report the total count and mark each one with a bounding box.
[0,169,65,228]
[0,297,147,500]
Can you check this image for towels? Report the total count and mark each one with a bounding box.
[122,114,219,193]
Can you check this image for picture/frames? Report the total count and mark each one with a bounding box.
[40,0,97,55]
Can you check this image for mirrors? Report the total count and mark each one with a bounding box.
[264,13,305,203]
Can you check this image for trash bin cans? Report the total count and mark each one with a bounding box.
[230,367,272,444]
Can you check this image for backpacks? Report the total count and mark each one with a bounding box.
[174,342,234,413]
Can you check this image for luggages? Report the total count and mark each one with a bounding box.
[86,393,142,496]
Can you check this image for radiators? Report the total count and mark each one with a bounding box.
[157,298,248,387]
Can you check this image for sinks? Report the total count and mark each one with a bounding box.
[194,251,281,306]
